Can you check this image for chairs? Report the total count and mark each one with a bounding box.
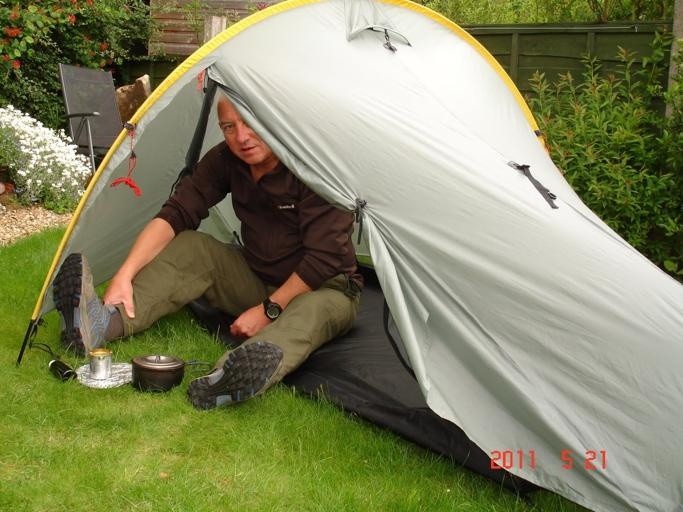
[61,62,124,174]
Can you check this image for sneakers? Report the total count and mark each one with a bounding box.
[186,343,284,410]
[52,253,118,359]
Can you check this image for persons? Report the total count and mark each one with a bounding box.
[52,94,365,411]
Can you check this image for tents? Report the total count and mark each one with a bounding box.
[16,0,683,511]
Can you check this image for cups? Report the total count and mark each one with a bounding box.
[88,347,113,382]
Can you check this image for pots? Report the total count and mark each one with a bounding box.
[130,353,186,392]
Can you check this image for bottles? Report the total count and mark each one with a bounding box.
[47,358,79,383]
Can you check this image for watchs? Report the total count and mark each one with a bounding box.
[263,297,282,320]
